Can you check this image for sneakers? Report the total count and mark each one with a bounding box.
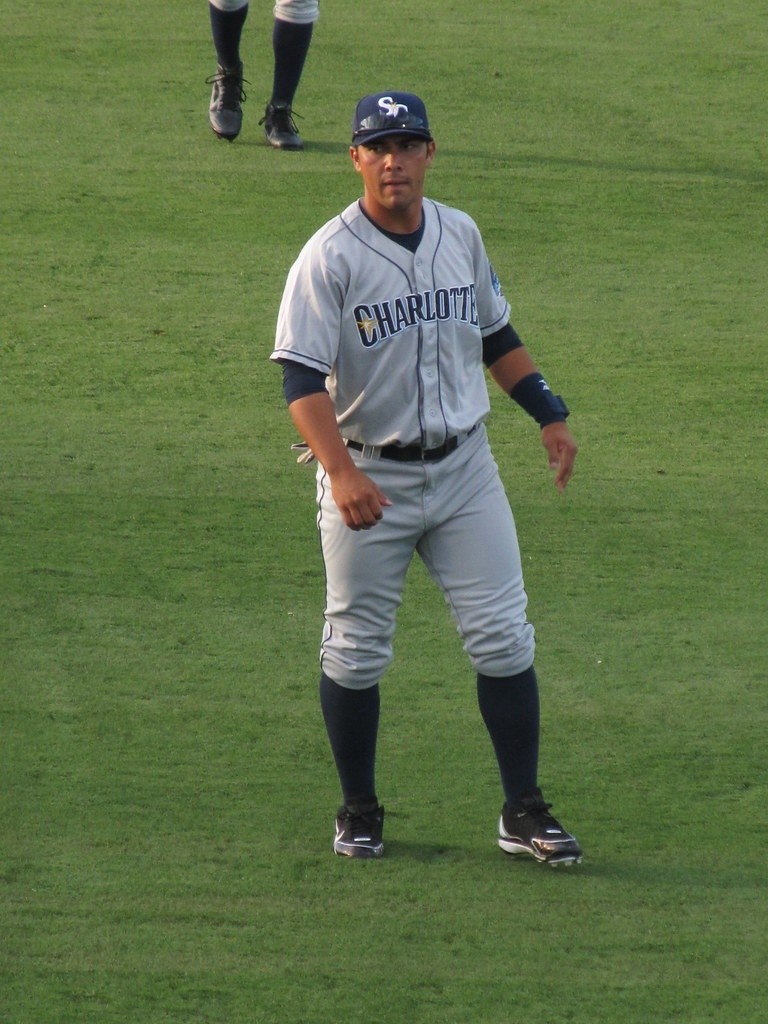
[497,789,583,867]
[264,105,305,149]
[332,800,386,858]
[209,63,244,140]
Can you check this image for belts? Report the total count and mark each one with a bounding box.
[345,424,477,462]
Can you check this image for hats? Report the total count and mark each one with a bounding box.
[353,92,430,145]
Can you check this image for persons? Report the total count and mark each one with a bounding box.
[270,91,582,867]
[209,0,319,150]
